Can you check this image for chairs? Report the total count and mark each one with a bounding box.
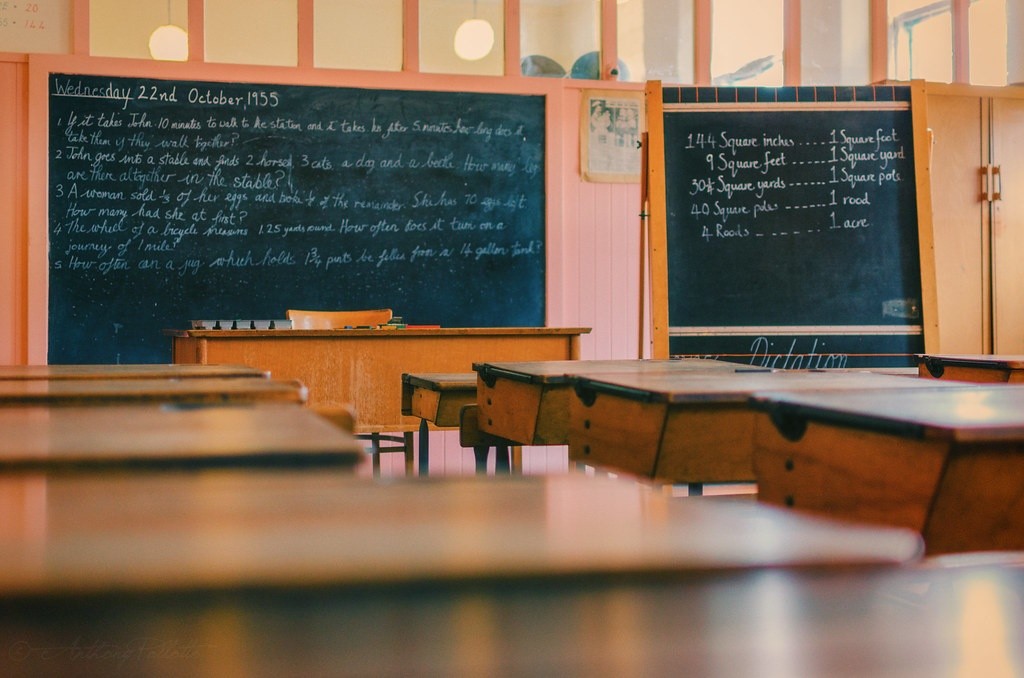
[461,405,523,476]
[286,309,414,478]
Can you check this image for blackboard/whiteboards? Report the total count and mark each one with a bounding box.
[27,53,564,366]
[644,79,940,372]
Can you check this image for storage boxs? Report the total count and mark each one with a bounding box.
[190,320,292,330]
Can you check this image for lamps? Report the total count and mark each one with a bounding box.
[454,0,495,61]
[148,0,189,61]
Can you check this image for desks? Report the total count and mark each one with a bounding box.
[160,327,592,475]
[0,356,1023,678]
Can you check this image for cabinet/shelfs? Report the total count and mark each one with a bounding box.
[924,83,1024,356]
